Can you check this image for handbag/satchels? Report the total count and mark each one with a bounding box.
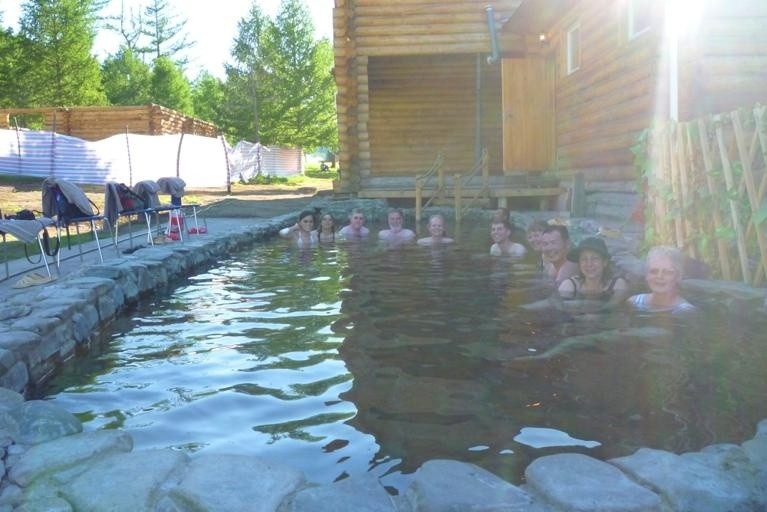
[115,183,144,212]
[54,186,87,218]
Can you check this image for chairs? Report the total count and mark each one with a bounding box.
[1,184,208,279]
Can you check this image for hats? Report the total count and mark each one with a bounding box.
[566,237,612,263]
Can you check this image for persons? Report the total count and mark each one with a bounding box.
[312,213,340,240]
[378,210,413,239]
[491,207,509,221]
[279,210,318,242]
[541,224,581,284]
[624,245,698,327]
[489,219,527,257]
[528,219,555,279]
[320,161,329,171]
[415,214,455,244]
[338,207,370,235]
[557,236,629,312]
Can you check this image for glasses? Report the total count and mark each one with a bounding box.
[646,267,676,276]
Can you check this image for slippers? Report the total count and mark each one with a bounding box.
[122,244,146,254]
[148,233,180,245]
[188,225,207,233]
[12,270,61,289]
